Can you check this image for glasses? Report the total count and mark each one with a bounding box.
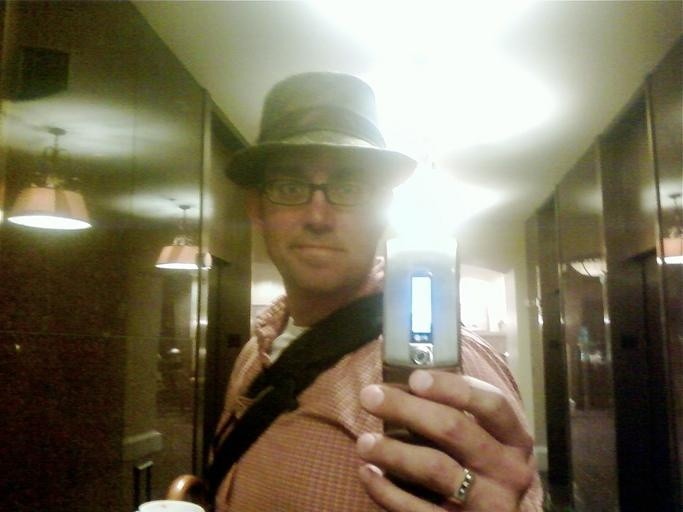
[258,178,371,206]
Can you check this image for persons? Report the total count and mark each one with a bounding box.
[204,71,546,512]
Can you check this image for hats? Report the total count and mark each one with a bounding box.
[224,72,417,188]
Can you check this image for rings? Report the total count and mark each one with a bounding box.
[452,466,475,508]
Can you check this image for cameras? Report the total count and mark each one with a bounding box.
[382,238,461,503]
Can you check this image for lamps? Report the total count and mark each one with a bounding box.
[153,204,213,271]
[7,127,92,231]
